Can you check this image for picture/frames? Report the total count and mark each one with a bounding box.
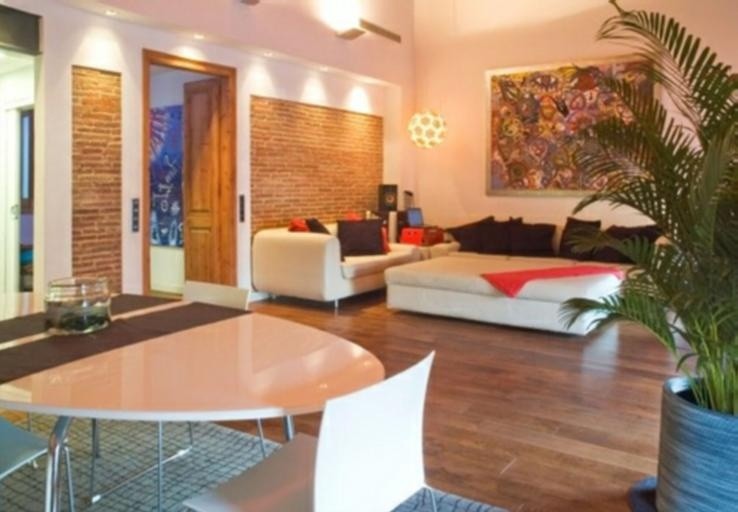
[485,52,661,197]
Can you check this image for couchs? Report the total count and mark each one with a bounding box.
[253,223,422,308]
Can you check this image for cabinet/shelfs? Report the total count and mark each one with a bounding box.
[374,211,409,243]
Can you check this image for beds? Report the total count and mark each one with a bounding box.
[385,226,668,336]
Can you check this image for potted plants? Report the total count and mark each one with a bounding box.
[558,0,738,512]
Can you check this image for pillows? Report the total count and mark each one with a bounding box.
[444,215,663,263]
[288,210,391,261]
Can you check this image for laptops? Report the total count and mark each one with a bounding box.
[407,208,437,228]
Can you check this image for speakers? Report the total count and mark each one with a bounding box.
[378,184,397,211]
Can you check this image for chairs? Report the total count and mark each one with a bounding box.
[0,416,76,512]
[183,350,438,512]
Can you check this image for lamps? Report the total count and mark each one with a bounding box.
[407,109,447,147]
